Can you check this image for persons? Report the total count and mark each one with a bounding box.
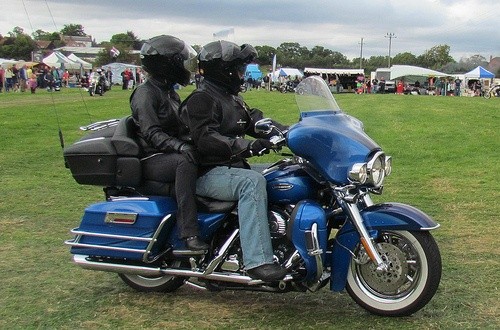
[0,64,113,94]
[241,74,299,93]
[472,81,482,95]
[455,77,461,96]
[415,81,419,87]
[356,77,385,94]
[121,67,142,89]
[397,80,404,94]
[129,36,211,252]
[177,40,287,284]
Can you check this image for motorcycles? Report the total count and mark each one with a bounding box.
[63,77,443,318]
[87,72,111,97]
[280,80,304,95]
[483,83,500,100]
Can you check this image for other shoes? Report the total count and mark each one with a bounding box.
[183,235,209,251]
[247,263,288,281]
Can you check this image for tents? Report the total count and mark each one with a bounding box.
[278,69,302,79]
[463,66,495,83]
[102,62,138,85]
[42,52,92,73]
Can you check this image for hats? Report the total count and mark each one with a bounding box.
[97,69,102,72]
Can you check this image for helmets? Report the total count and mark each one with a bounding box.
[197,40,259,97]
[140,34,192,87]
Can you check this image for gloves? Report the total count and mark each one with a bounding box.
[247,139,275,157]
[178,143,203,165]
